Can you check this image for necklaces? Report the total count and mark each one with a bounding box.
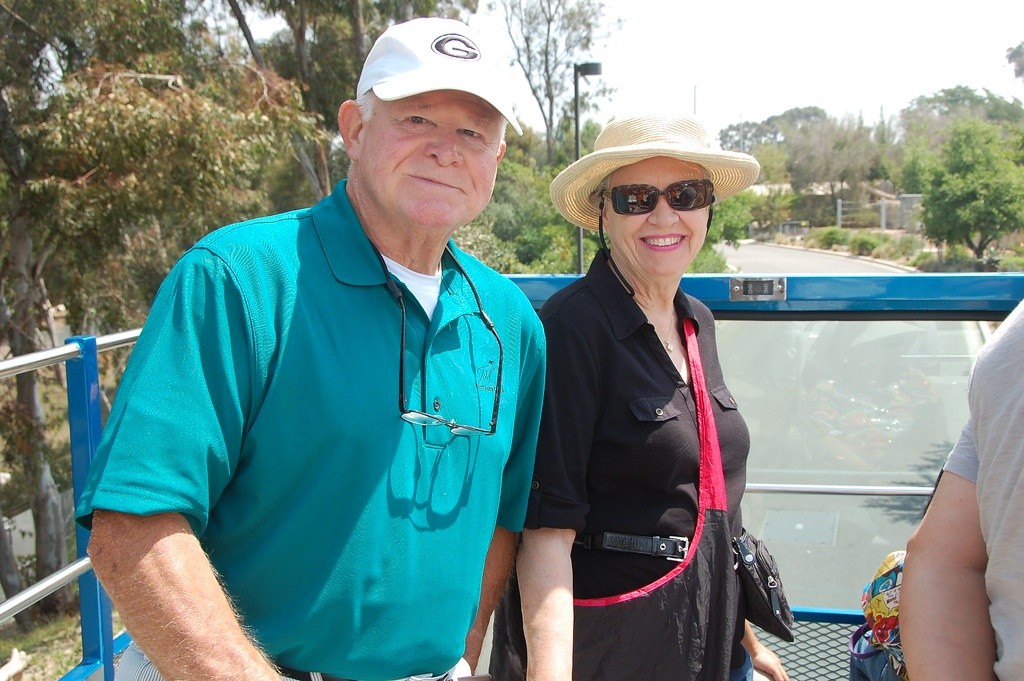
[656,319,674,352]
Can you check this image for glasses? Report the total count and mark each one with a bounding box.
[388,279,503,436]
[598,178,715,215]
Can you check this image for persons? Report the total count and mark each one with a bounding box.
[73,17,547,681]
[487,113,791,681]
[901,299,1024,680]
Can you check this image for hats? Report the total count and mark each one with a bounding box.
[355,17,525,137]
[549,103,759,231]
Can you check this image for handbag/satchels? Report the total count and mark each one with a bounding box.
[504,497,733,681]
[732,526,796,642]
[850,550,909,681]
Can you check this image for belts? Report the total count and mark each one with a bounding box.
[275,665,358,681]
[578,532,692,563]
